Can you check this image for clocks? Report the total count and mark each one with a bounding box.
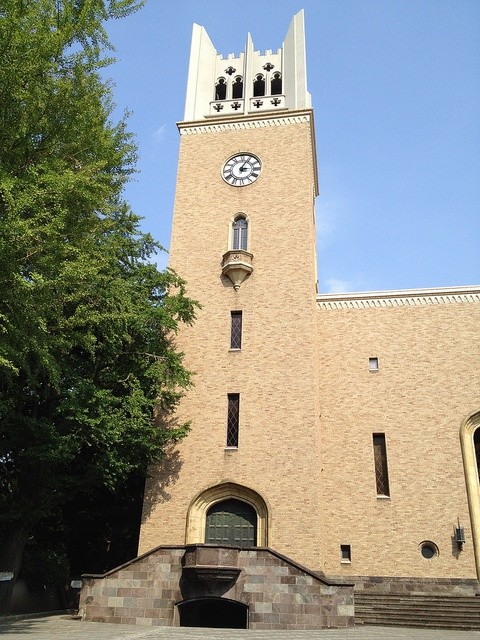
[221,151,263,187]
